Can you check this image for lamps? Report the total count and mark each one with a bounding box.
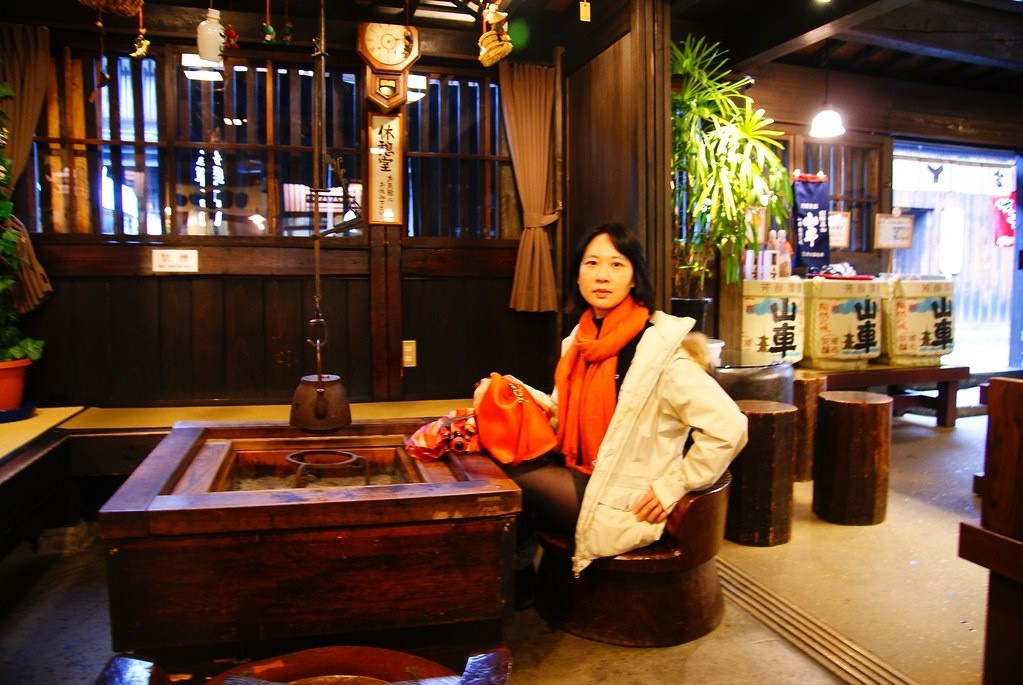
[809,38,845,138]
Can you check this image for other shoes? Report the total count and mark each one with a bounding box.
[513,567,540,613]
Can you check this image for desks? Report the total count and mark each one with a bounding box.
[819,367,971,428]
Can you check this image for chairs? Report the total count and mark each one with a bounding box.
[533,469,733,647]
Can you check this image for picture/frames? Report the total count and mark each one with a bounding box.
[745,207,768,244]
[368,112,403,225]
[874,214,914,249]
[828,211,850,247]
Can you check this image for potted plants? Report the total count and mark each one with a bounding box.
[0,78,45,412]
[669,33,793,333]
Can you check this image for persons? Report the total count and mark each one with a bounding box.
[473,223,749,610]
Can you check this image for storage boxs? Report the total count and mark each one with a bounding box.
[742,278,955,371]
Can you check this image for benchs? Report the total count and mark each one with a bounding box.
[56,399,475,477]
[1,407,85,500]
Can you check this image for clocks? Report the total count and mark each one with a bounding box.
[355,22,422,114]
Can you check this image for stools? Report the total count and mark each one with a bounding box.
[724,400,798,548]
[794,370,826,482]
[812,391,894,526]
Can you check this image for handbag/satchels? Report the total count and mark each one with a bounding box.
[474,371,559,468]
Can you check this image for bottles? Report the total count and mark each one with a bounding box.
[775,230,792,277]
[765,229,777,251]
